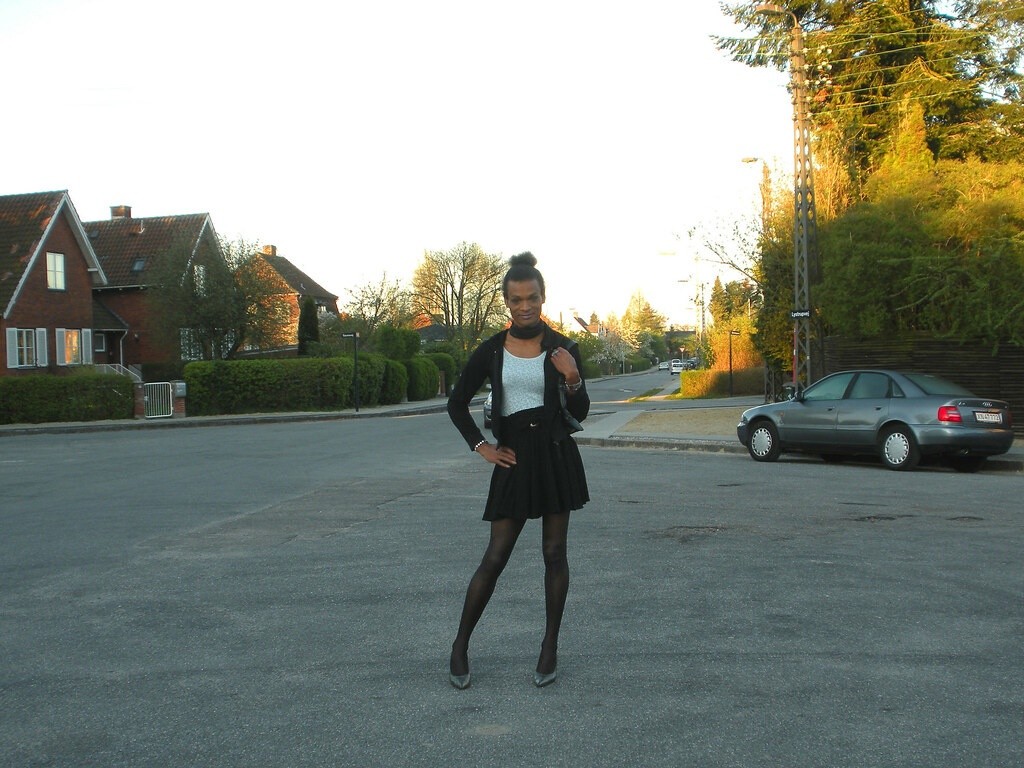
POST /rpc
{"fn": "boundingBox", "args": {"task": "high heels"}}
[534,646,557,686]
[449,647,470,688]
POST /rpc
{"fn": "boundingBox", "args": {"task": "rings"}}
[551,352,556,356]
[555,350,559,354]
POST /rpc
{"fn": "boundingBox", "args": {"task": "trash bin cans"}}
[781,382,795,401]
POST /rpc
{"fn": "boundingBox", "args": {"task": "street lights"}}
[755,5,816,385]
[729,330,741,397]
[677,279,706,369]
[342,332,359,412]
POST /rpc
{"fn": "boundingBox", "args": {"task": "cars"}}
[737,367,1015,469]
[659,361,669,371]
[484,384,494,430]
[671,356,701,375]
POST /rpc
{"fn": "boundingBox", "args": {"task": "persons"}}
[446,252,592,689]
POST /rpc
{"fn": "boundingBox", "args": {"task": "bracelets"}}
[474,440,486,451]
[565,377,582,392]
